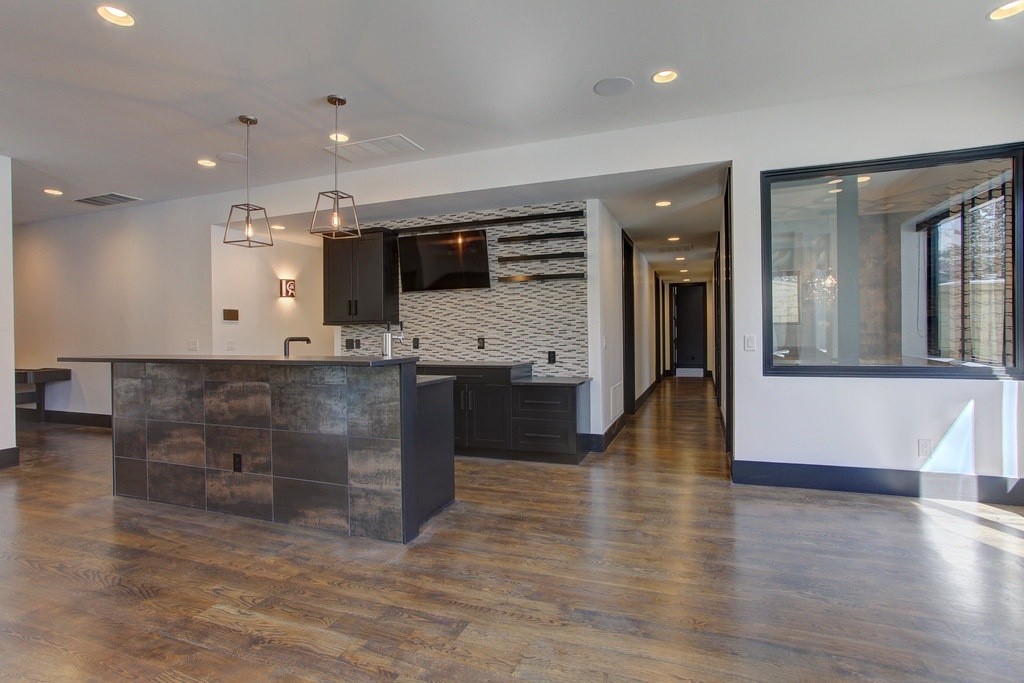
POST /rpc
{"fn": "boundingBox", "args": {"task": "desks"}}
[15,368,72,421]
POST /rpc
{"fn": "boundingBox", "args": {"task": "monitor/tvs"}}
[398,229,491,292]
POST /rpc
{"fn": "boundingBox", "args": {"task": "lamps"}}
[310,94,361,241]
[222,115,273,249]
[279,279,296,298]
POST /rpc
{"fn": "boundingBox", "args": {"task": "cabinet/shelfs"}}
[322,227,400,326]
[417,360,594,465]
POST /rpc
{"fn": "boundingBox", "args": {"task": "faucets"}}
[381,331,405,356]
[284,336,312,355]
